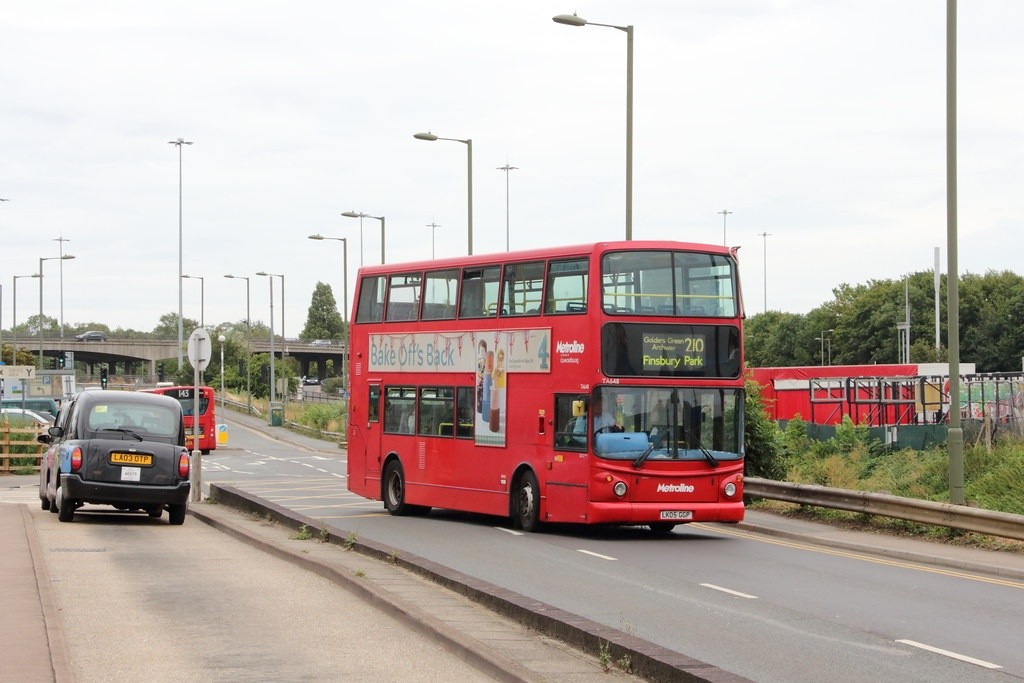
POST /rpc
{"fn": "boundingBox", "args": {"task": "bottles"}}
[538,409,545,434]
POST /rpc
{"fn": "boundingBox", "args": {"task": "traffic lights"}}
[49,357,57,370]
[59,350,66,367]
[156,364,163,381]
[100,367,108,387]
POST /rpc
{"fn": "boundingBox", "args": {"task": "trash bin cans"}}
[270,401,284,427]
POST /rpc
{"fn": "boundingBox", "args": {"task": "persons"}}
[374,392,392,420]
[408,401,428,434]
[572,395,624,447]
[442,406,472,437]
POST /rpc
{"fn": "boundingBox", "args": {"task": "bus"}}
[346,239,749,534]
[134,381,217,455]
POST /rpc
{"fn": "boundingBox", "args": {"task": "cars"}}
[311,340,332,348]
[49,390,191,526]
[75,331,107,343]
[303,377,319,385]
[0,399,60,440]
[36,400,73,512]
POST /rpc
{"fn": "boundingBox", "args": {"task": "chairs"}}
[376,302,705,321]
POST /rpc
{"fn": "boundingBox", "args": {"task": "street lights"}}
[413,131,476,256]
[341,211,386,305]
[551,13,634,308]
[224,274,253,417]
[181,273,205,329]
[168,135,194,377]
[255,272,286,401]
[218,336,226,417]
[39,254,75,371]
[495,163,521,253]
[53,237,70,340]
[814,337,830,366]
[424,221,441,258]
[308,235,349,399]
[820,329,835,366]
[11,272,45,366]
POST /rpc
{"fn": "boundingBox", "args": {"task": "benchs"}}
[399,404,413,433]
[430,406,448,435]
